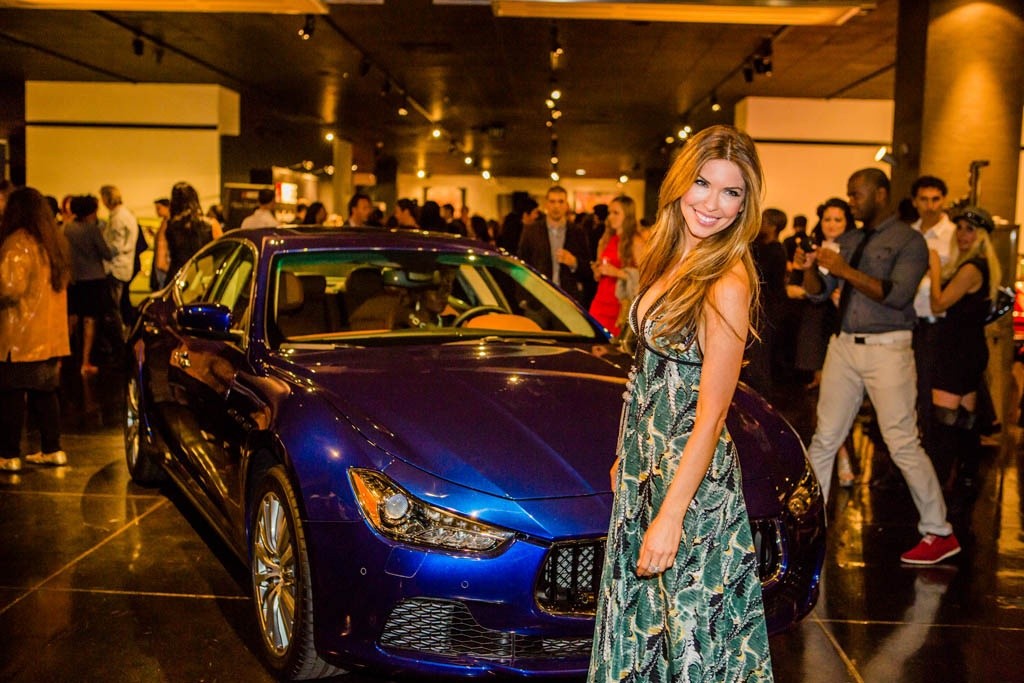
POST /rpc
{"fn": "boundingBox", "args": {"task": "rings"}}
[648,566,658,573]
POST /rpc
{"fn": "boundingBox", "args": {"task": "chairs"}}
[278,267,385,337]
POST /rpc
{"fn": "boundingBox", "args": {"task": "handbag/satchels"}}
[982,285,1017,330]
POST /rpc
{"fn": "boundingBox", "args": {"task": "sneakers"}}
[899,534,962,565]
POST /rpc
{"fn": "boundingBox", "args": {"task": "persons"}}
[0,167,1024,565]
[583,124,775,683]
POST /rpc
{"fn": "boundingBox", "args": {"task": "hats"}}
[817,198,854,228]
[950,206,994,234]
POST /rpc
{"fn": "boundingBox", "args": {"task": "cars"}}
[120,225,825,683]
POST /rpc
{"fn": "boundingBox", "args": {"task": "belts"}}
[852,338,867,344]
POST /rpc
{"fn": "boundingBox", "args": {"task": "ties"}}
[832,232,874,339]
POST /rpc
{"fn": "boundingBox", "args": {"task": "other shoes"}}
[0,456,23,474]
[23,450,68,467]
[79,364,99,377]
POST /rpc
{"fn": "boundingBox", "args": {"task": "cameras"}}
[800,238,815,253]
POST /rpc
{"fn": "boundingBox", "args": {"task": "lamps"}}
[743,39,773,83]
[876,147,898,167]
